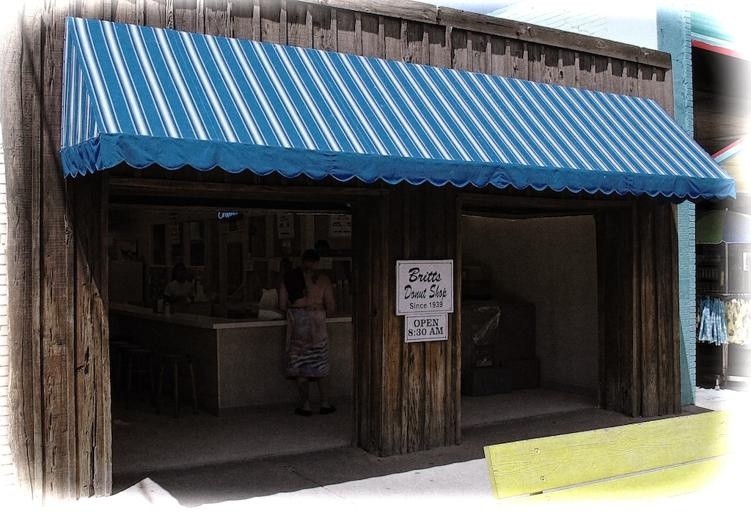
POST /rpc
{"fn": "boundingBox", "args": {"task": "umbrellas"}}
[694,206,751,368]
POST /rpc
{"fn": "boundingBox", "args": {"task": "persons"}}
[314,239,346,288]
[278,247,339,419]
[163,262,196,304]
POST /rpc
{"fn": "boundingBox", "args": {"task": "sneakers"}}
[320,403,338,414]
[294,404,313,416]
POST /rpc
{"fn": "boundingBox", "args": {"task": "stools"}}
[110,337,200,419]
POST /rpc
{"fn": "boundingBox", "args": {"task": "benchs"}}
[483,410,728,501]
